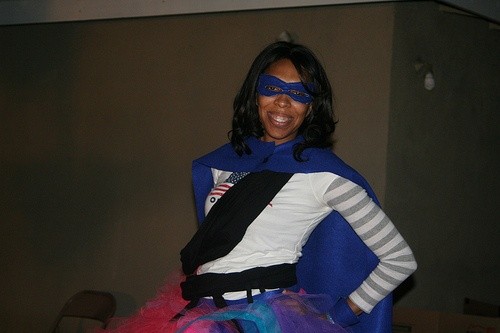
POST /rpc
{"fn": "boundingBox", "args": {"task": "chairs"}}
[49,289,117,333]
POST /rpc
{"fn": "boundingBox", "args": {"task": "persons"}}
[178,39,417,333]
